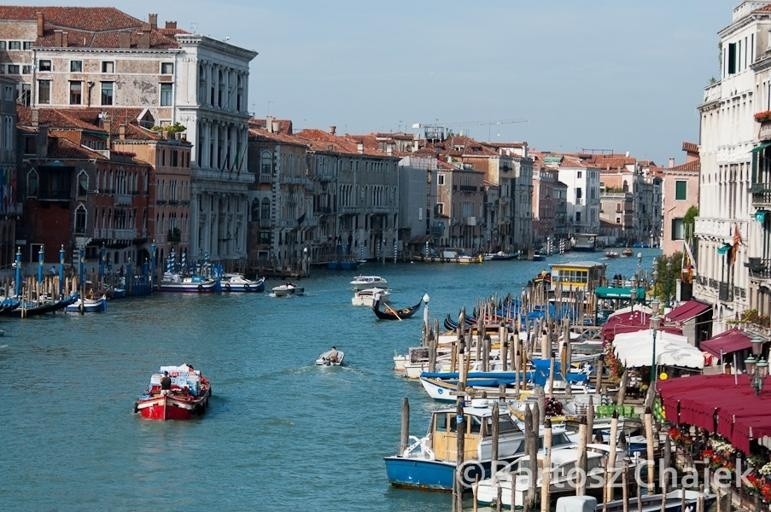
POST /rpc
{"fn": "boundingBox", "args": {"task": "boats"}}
[328,259,367,270]
[153,272,265,293]
[606,248,619,257]
[457,251,545,261]
[622,248,632,256]
[0,280,126,313]
[271,286,304,296]
[135,362,211,422]
[315,350,344,366]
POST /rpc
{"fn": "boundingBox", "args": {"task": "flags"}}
[680,223,744,284]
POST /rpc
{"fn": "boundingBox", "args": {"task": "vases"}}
[758,123,771,142]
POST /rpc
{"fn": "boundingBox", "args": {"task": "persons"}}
[327,346,339,363]
[160,370,171,398]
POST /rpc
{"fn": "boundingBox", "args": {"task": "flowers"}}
[754,111,771,123]
